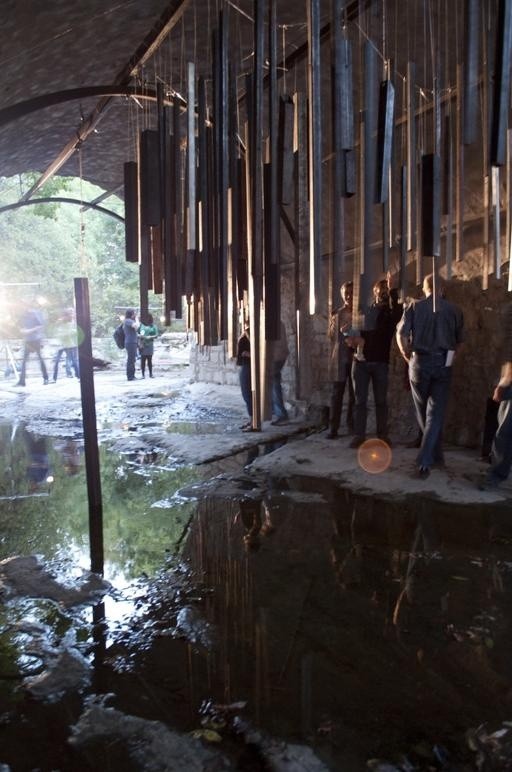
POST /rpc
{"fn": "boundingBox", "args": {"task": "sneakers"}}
[355,353,366,361]
[476,457,491,464]
[419,457,444,479]
[271,416,289,425]
[326,428,423,448]
[239,422,261,432]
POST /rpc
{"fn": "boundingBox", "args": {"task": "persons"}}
[13,303,50,387]
[395,271,465,479]
[462,293,512,489]
[136,311,157,379]
[325,270,405,451]
[122,307,143,380]
[237,308,289,433]
[49,307,81,385]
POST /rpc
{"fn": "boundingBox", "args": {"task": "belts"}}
[412,351,432,356]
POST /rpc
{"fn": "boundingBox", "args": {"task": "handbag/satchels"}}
[114,323,125,349]
